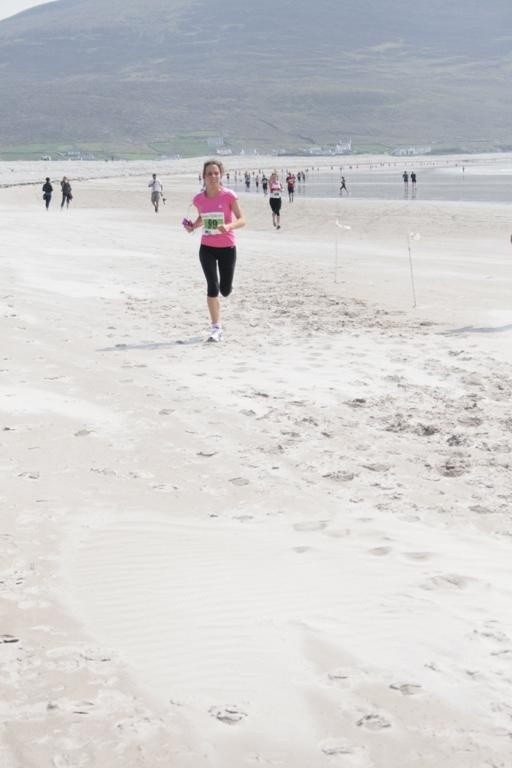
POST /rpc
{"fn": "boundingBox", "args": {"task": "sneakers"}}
[208,327,223,342]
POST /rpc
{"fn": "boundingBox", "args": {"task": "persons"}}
[184,160,247,342]
[42,175,54,210]
[59,174,72,210]
[148,172,164,213]
[197,166,417,232]
